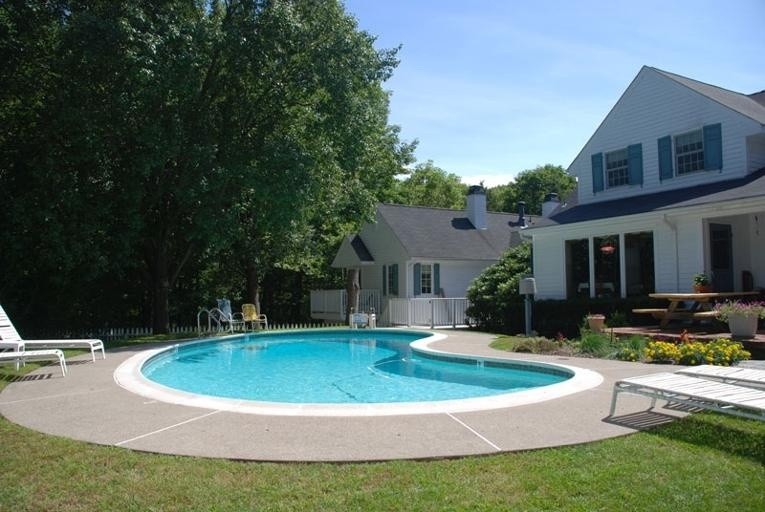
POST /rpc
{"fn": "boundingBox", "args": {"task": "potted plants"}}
[693,270,715,293]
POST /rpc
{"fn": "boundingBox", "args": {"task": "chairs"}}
[217,299,246,334]
[0,304,106,363]
[0,349,67,377]
[675,365,765,388]
[609,373,765,423]
[242,304,268,331]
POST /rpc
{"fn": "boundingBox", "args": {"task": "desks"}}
[648,291,760,327]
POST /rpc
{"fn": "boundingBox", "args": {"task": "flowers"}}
[713,299,765,323]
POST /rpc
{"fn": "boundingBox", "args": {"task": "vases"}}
[728,312,759,338]
[588,314,606,330]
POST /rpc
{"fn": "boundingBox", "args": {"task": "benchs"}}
[632,308,690,327]
[694,311,716,319]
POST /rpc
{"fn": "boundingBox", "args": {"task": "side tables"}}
[0,340,25,371]
